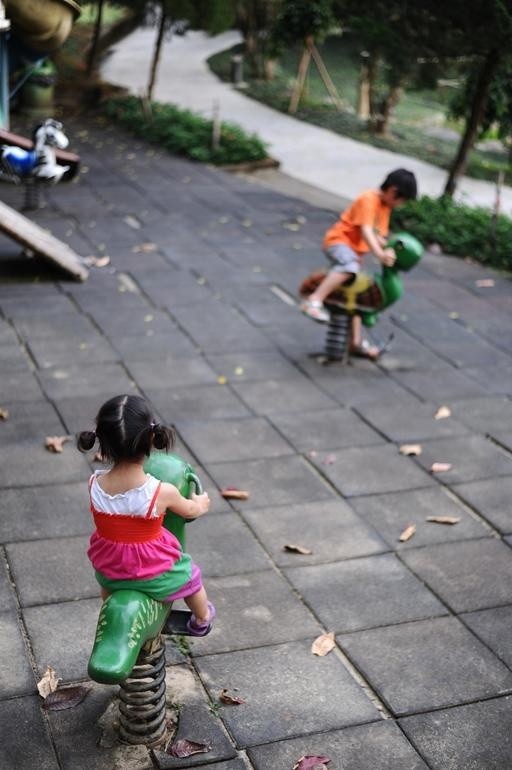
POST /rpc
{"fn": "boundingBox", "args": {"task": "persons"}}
[298,168,418,360]
[76,392,217,637]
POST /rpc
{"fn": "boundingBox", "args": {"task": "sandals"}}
[299,298,331,324]
[350,338,379,359]
[187,600,216,636]
